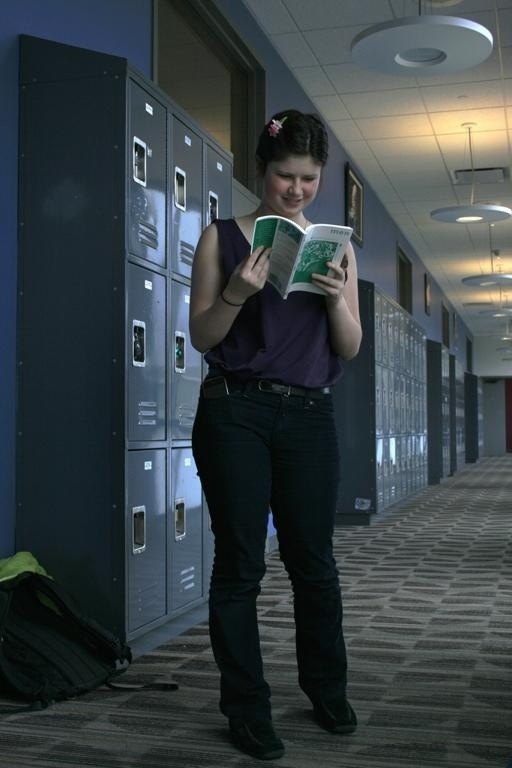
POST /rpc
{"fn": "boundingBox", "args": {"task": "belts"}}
[236,376,330,403]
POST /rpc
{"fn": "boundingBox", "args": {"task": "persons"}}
[189,110,361,761]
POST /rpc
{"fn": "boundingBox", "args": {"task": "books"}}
[249,216,352,299]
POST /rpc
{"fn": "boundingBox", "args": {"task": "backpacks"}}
[0,551,133,711]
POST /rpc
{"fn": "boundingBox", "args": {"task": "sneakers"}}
[313,701,358,734]
[229,720,285,761]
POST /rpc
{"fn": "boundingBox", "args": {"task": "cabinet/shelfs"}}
[12,30,237,645]
[331,279,485,518]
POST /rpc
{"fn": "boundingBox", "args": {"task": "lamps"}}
[428,120,511,321]
[349,1,497,80]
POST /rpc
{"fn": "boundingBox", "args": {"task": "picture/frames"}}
[343,160,364,249]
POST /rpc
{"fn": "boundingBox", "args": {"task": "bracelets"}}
[220,291,247,307]
